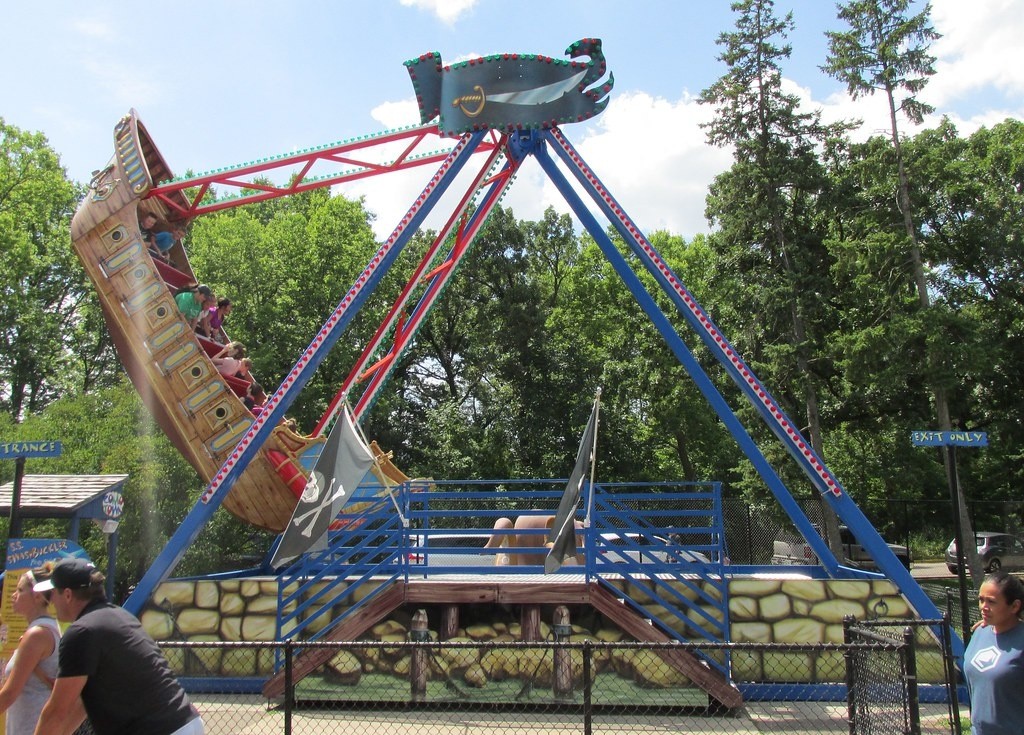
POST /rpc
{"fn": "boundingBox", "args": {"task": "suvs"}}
[769,525,912,573]
[592,532,710,564]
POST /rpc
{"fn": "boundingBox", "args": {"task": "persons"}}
[717,551,729,565]
[665,525,681,564]
[33,557,203,735]
[963,570,1024,735]
[195,297,231,339]
[211,341,246,377]
[239,382,264,411]
[151,225,187,261]
[252,394,273,415]
[197,293,215,343]
[139,212,158,247]
[234,358,252,380]
[0,561,62,735]
[173,282,211,332]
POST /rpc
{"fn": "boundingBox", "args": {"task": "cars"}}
[944,531,1024,574]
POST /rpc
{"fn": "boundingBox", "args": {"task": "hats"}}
[33,557,103,592]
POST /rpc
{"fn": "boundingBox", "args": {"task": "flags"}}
[544,398,601,574]
[270,404,375,570]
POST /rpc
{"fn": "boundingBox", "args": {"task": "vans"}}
[392,532,501,567]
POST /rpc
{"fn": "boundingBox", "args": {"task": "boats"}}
[71,107,434,535]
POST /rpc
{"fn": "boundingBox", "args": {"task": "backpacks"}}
[33,624,94,735]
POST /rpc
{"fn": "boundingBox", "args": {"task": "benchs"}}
[152,255,265,420]
